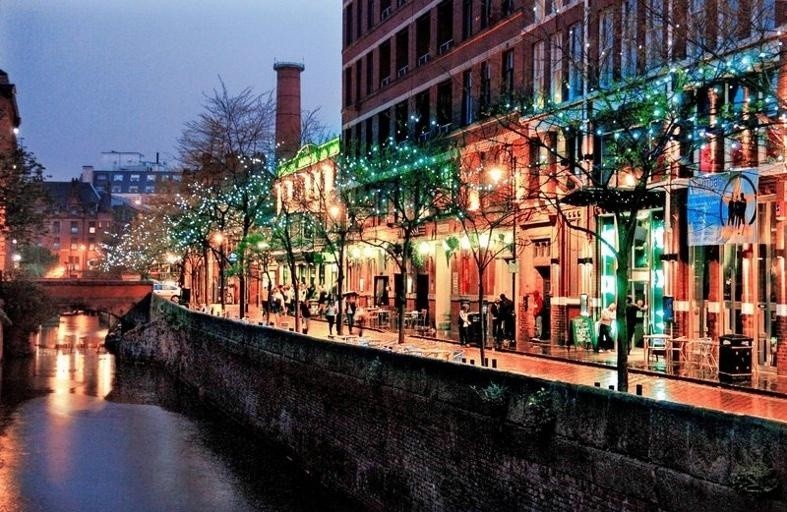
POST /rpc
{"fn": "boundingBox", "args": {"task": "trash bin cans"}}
[465,312,487,347]
[180,288,191,309]
[718,334,754,388]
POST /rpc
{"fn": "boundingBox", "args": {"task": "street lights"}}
[80,244,86,275]
[490,149,517,350]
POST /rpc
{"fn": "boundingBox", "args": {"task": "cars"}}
[153,283,182,304]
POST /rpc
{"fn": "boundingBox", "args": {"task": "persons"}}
[258,279,312,334]
[316,291,366,338]
[491,294,515,346]
[458,299,489,348]
[723,190,746,236]
[380,290,389,324]
[593,297,648,354]
[532,290,544,341]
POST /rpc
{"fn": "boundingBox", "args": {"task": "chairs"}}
[364,307,427,332]
[345,336,464,364]
[643,334,759,382]
